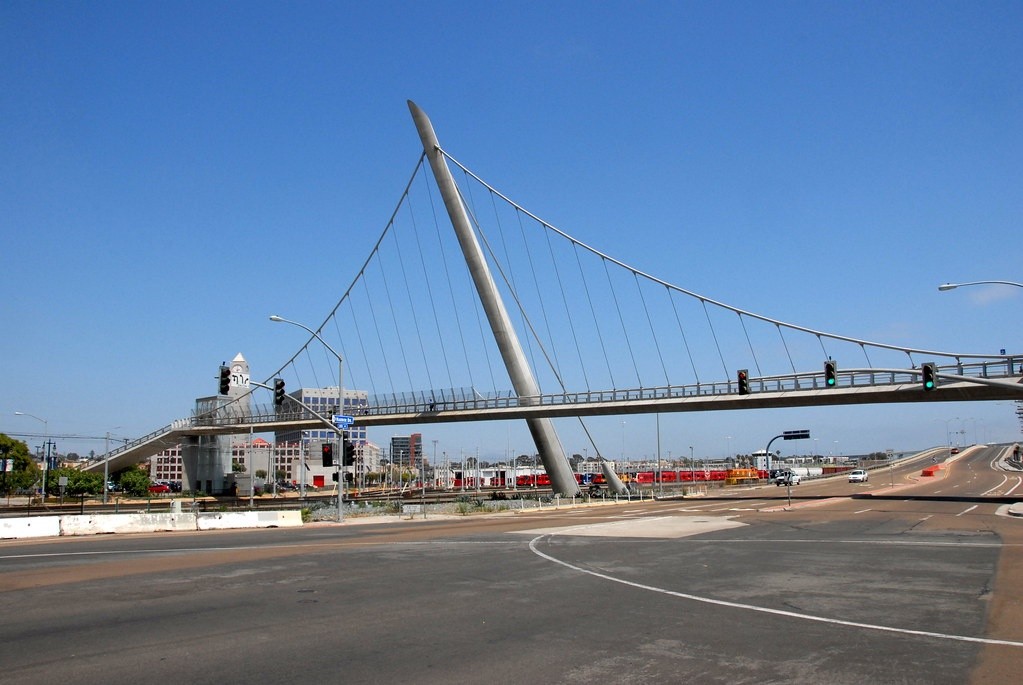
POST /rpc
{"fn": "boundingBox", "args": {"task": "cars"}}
[775,471,801,486]
[951,448,959,453]
[848,470,868,483]
[168,483,182,493]
[275,481,318,491]
[146,482,170,492]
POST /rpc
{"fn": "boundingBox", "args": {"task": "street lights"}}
[269,315,345,521]
[14,411,48,504]
[934,417,959,455]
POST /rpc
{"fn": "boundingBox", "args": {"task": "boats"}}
[737,369,750,394]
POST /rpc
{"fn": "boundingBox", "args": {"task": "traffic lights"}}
[274,380,286,405]
[922,362,937,392]
[218,366,231,397]
[824,360,838,388]
